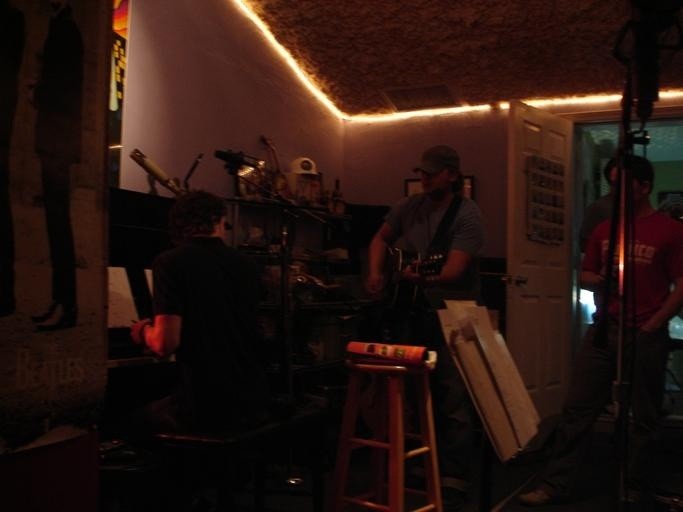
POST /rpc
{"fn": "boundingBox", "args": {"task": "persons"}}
[517,154,682,505]
[24,0,85,332]
[0,6,27,318]
[128,189,272,448]
[364,145,484,511]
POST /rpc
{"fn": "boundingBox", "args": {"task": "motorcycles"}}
[489,129,682,512]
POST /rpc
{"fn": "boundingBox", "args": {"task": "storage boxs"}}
[154,390,331,511]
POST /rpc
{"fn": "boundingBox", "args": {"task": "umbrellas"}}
[33,301,77,330]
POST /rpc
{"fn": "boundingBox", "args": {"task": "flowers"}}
[131,149,178,194]
[260,134,282,175]
[625,0,664,122]
[214,150,256,167]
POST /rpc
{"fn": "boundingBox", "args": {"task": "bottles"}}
[412,145,460,176]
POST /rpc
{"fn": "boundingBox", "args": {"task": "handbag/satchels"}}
[138,322,151,339]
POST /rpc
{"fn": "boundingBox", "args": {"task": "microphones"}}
[330,350,443,512]
